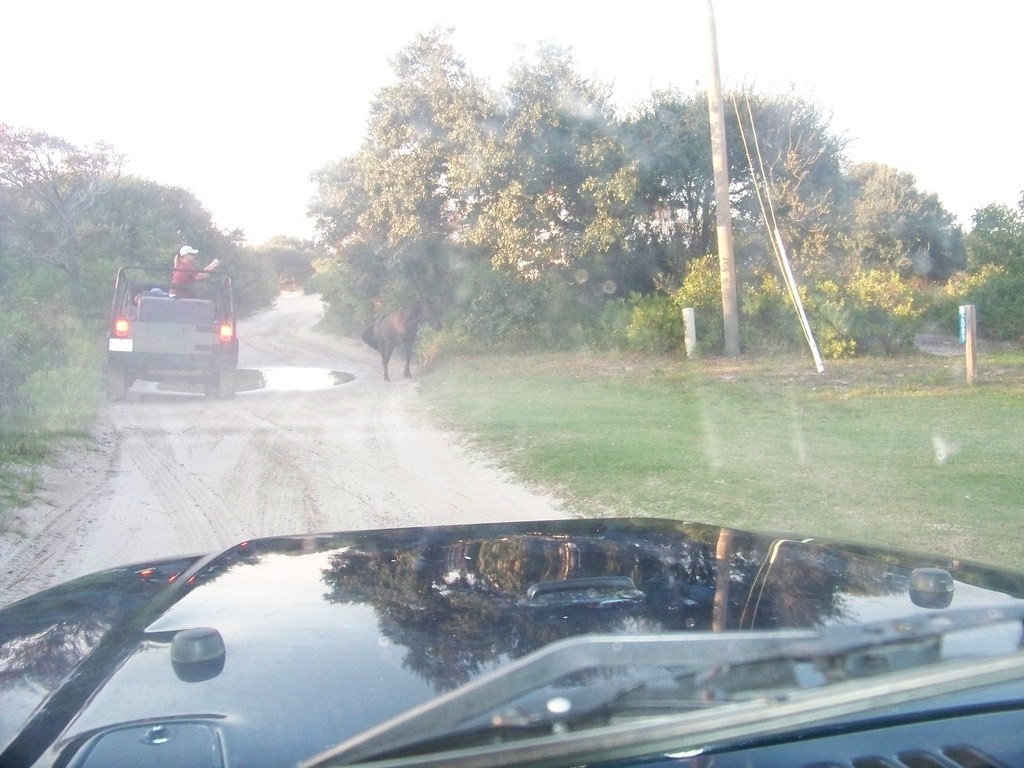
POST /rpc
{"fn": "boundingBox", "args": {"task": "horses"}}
[363,298,442,381]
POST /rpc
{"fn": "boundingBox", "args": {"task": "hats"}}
[151,288,169,297]
[180,246,199,256]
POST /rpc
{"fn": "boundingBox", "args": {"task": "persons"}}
[168,246,214,297]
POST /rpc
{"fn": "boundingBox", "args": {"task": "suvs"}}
[106,266,240,401]
